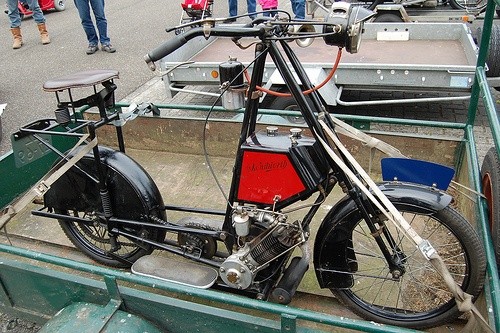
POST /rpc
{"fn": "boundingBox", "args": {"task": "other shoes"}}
[223,18,236,23]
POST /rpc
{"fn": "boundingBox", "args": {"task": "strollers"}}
[174,0,215,36]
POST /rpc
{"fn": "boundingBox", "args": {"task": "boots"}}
[37,21,51,45]
[10,26,24,49]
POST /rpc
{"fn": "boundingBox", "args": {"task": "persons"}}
[74,0,117,54]
[6,0,51,49]
[223,0,258,24]
[257,0,279,21]
[290,0,306,20]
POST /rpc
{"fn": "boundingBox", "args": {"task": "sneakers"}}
[86,44,99,54]
[101,42,116,53]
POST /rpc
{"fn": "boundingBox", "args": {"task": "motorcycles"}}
[10,0,488,331]
